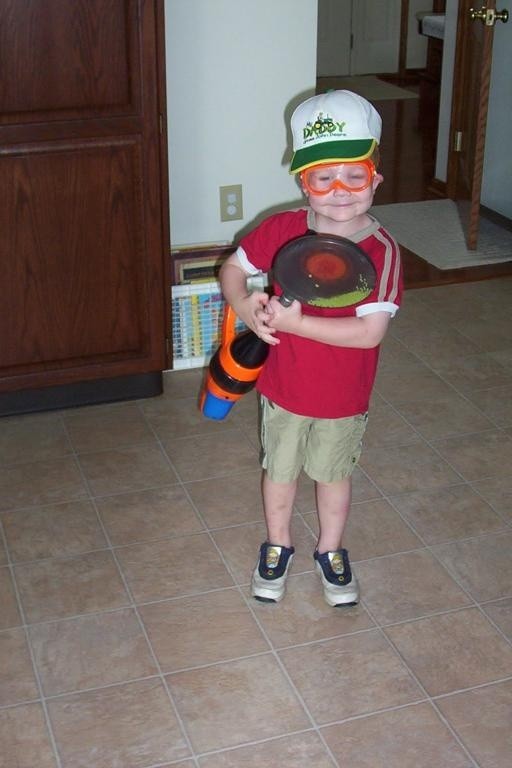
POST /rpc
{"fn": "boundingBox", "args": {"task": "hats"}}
[288,89,383,175]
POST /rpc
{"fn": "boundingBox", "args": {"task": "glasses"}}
[299,160,376,195]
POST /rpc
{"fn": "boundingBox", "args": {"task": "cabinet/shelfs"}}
[0,0,173,418]
[317,1,406,79]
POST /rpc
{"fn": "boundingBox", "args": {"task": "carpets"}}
[366,197,512,270]
[315,73,421,103]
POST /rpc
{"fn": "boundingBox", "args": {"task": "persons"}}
[215,89,403,610]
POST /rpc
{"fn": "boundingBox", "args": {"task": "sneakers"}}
[251,544,294,602]
[314,545,360,608]
